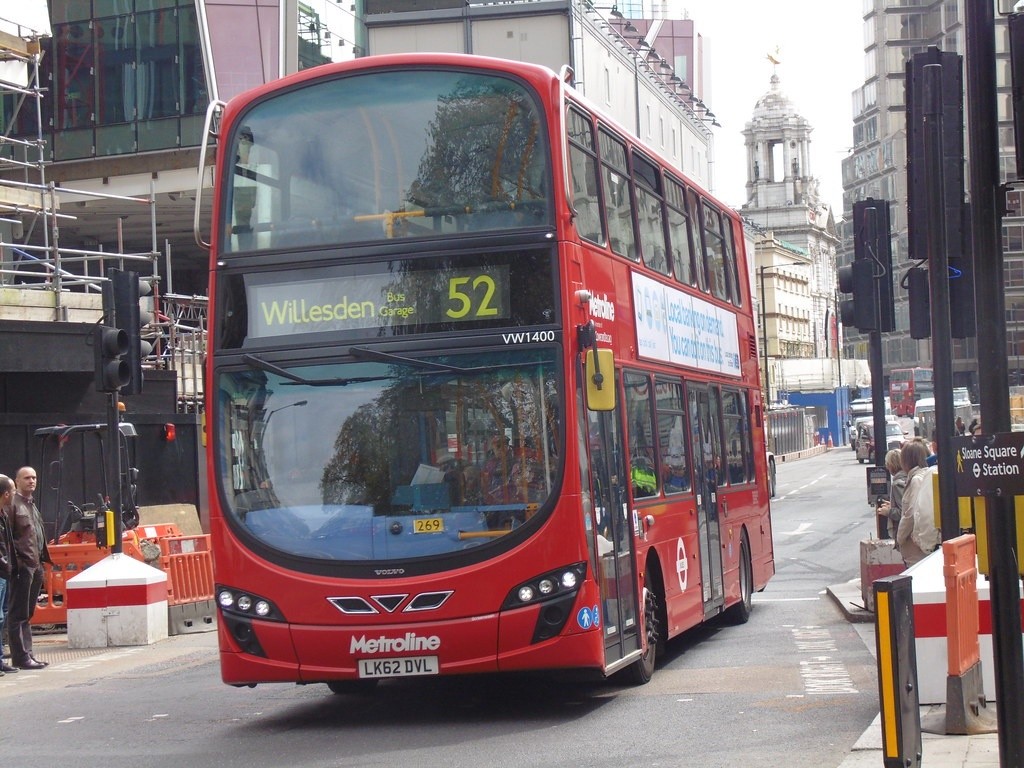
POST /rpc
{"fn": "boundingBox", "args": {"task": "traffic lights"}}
[94,324,132,391]
[837,260,859,329]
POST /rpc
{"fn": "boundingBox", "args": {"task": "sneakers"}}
[0,660,19,677]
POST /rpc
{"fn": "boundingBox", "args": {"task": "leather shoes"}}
[12,657,50,669]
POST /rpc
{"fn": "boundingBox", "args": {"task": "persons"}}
[876,416,982,565]
[0,466,51,678]
[593,443,745,557]
[457,436,557,530]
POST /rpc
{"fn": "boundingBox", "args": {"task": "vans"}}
[849,387,974,464]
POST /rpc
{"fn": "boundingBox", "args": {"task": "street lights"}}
[114,267,153,395]
[260,399,309,451]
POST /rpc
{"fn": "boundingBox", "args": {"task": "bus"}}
[889,367,934,419]
[192,52,776,696]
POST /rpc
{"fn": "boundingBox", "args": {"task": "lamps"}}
[623,36,649,47]
[594,6,623,17]
[701,118,721,127]
[685,99,707,108]
[657,72,682,81]
[694,109,715,117]
[647,58,673,70]
[636,48,659,59]
[610,21,638,31]
[676,93,698,102]
[666,82,691,91]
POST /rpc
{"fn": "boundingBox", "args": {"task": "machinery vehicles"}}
[15,401,219,637]
